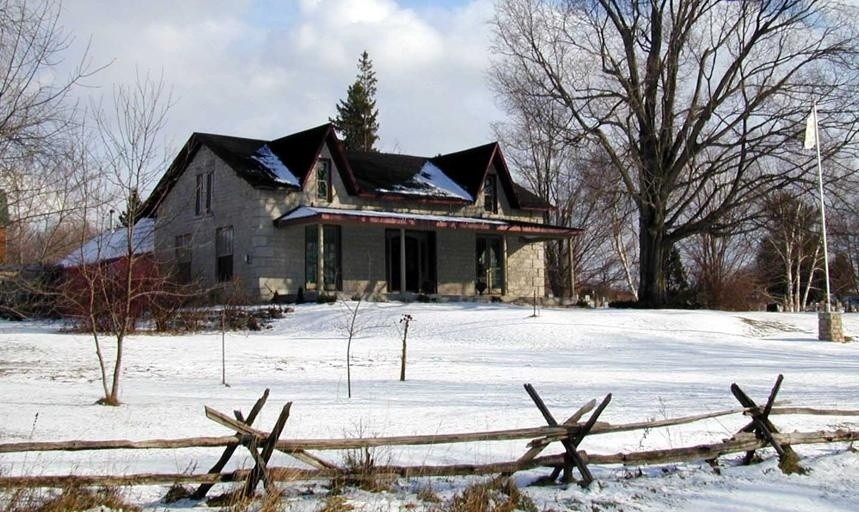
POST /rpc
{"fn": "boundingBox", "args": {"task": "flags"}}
[804,110,816,150]
